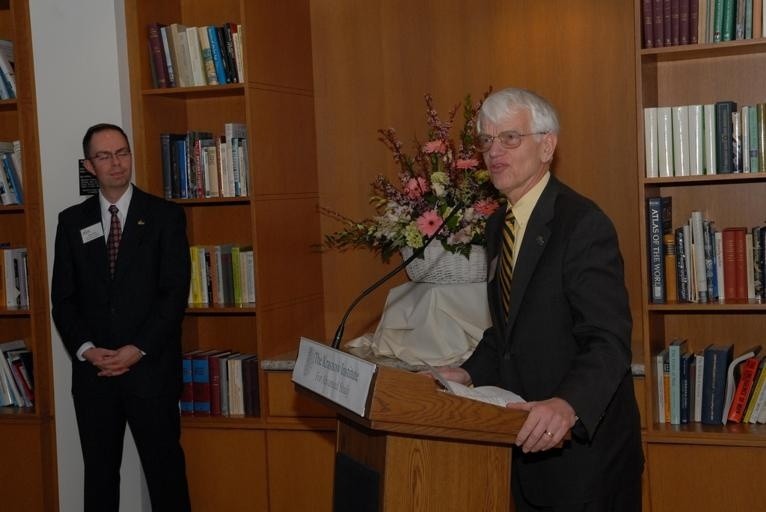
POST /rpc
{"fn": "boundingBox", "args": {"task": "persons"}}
[51,123,192,512]
[417,88,646,512]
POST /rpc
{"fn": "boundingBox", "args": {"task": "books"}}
[0,40,35,407]
[649,195,766,303]
[657,339,766,427]
[644,101,766,178]
[643,0,766,49]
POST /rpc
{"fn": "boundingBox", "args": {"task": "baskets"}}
[400,243,490,283]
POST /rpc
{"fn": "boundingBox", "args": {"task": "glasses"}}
[471,129,547,152]
[88,149,131,163]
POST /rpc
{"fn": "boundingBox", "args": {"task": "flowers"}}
[305,88,500,262]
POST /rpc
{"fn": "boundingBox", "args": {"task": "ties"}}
[106,205,122,279]
[499,207,516,322]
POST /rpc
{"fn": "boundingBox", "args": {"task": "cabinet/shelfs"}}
[630,3,766,437]
[0,1,59,509]
[114,2,317,422]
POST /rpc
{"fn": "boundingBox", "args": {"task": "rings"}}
[544,430,554,438]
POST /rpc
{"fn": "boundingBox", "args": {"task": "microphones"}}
[331,200,464,349]
